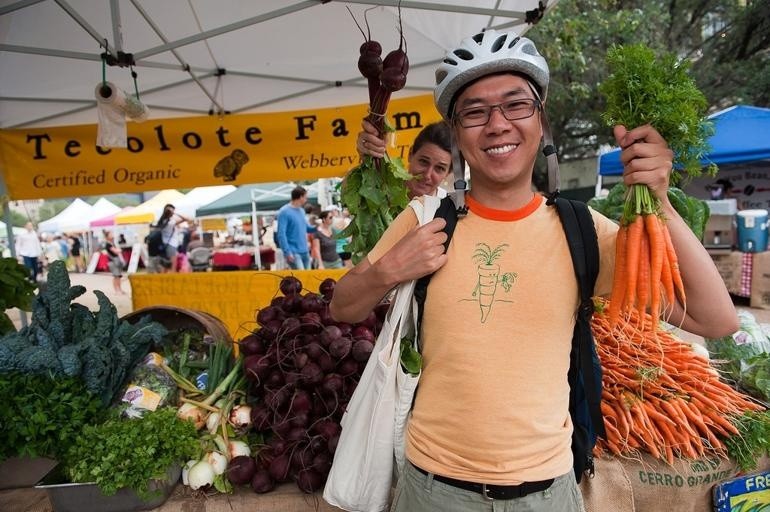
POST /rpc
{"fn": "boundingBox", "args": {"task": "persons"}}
[355,119,455,201]
[705,178,743,212]
[327,26,746,511]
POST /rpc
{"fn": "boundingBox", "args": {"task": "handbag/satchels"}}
[318,284,428,511]
[113,258,127,267]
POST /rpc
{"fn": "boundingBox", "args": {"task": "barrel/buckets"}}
[737,208,768,252]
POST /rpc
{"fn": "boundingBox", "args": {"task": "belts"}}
[404,455,556,498]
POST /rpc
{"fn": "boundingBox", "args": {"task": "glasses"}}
[454,97,534,128]
[327,215,333,220]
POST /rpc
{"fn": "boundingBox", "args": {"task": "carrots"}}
[476,263,499,322]
[610,218,686,342]
[224,276,376,496]
[592,297,767,464]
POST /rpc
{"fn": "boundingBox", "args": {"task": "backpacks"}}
[145,231,166,257]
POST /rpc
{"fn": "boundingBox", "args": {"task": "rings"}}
[362,139,366,147]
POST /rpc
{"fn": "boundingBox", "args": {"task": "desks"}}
[210,243,276,272]
[705,248,769,310]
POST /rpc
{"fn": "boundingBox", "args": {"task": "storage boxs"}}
[701,214,737,247]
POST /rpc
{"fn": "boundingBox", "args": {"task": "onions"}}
[176,403,253,492]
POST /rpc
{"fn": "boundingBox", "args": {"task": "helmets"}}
[432,28,551,116]
